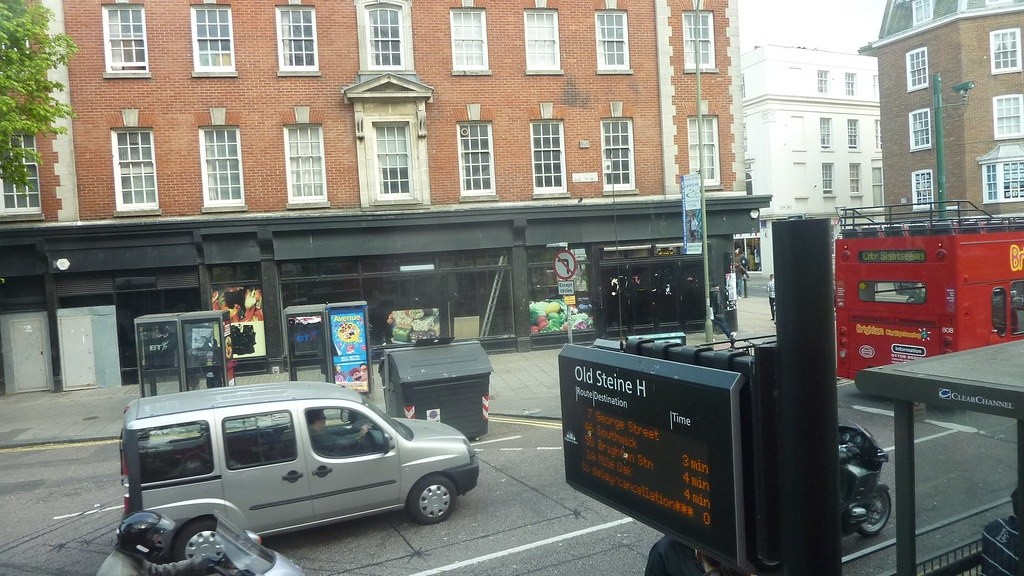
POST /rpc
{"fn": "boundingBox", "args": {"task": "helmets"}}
[116,511,176,557]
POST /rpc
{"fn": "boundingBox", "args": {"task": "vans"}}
[118,379,480,539]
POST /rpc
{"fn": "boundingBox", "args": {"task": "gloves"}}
[191,552,220,572]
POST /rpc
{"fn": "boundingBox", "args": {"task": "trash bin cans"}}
[378,338,492,440]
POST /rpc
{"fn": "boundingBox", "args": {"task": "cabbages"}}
[548,312,559,321]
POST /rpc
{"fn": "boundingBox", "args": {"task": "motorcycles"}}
[837,414,892,540]
[206,509,308,576]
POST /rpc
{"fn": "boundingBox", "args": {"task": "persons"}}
[709,283,737,341]
[198,333,223,387]
[767,274,777,321]
[645,532,744,576]
[735,262,750,299]
[310,410,369,456]
[96,510,225,576]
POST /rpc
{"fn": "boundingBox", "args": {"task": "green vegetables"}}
[530,298,590,334]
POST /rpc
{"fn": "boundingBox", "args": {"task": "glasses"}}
[315,416,327,420]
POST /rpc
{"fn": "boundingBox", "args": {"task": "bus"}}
[834,199,1024,380]
